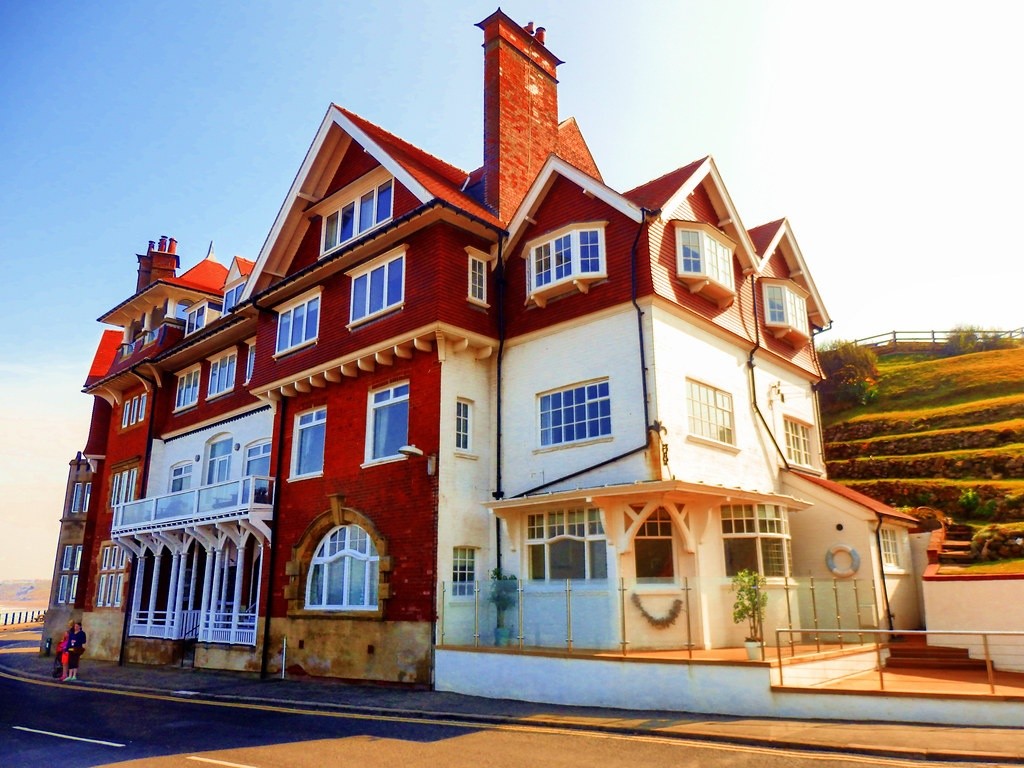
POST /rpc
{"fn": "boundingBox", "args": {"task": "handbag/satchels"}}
[73,646,85,655]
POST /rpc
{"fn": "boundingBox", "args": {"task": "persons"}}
[62,622,86,682]
[56,619,75,680]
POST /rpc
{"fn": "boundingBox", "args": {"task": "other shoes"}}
[72,676,77,680]
[63,677,72,681]
[57,675,66,680]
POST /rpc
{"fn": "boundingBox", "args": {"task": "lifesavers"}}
[825,543,861,578]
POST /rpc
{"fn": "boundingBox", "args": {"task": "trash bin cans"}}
[44,638,52,656]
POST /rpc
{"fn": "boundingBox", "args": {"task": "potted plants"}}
[491,568,519,647]
[731,570,768,659]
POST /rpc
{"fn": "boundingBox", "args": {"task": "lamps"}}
[398,446,436,476]
